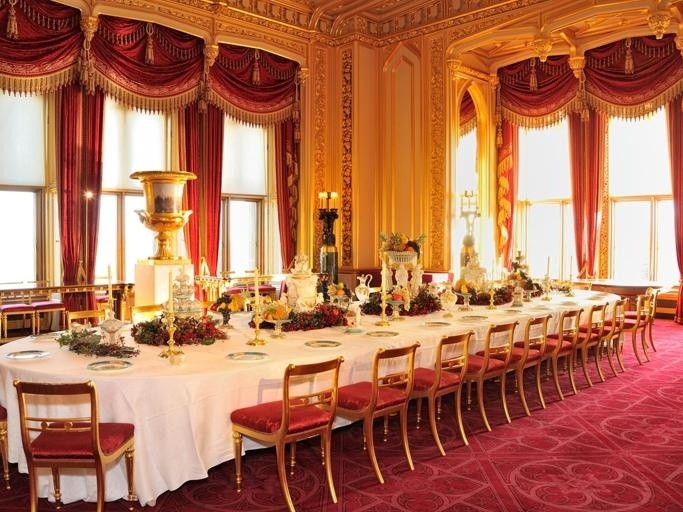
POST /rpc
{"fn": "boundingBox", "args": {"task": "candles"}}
[459,195,478,213]
[318,191,340,210]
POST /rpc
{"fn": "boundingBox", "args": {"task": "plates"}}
[86,360,133,374]
[5,350,50,360]
[366,331,400,337]
[459,316,488,322]
[423,322,452,329]
[226,352,272,363]
[305,340,343,350]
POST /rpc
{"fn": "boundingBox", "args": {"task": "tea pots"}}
[354,274,373,303]
